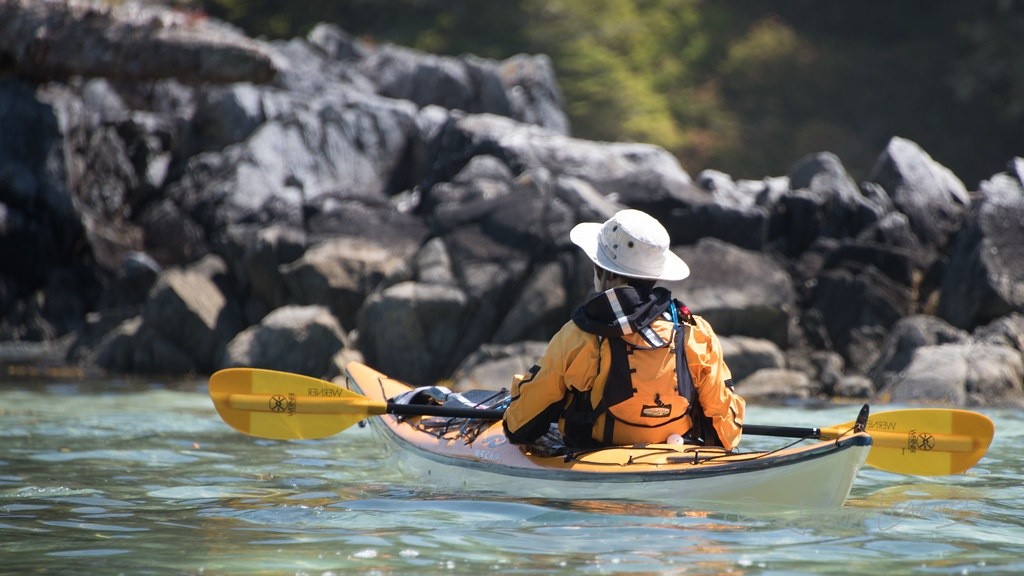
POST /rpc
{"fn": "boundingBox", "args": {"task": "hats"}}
[569,209,690,281]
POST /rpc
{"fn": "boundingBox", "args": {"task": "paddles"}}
[206,365,996,479]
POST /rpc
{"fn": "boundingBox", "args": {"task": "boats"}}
[341,361,870,512]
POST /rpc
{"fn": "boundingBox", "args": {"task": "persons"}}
[501,209,746,452]
